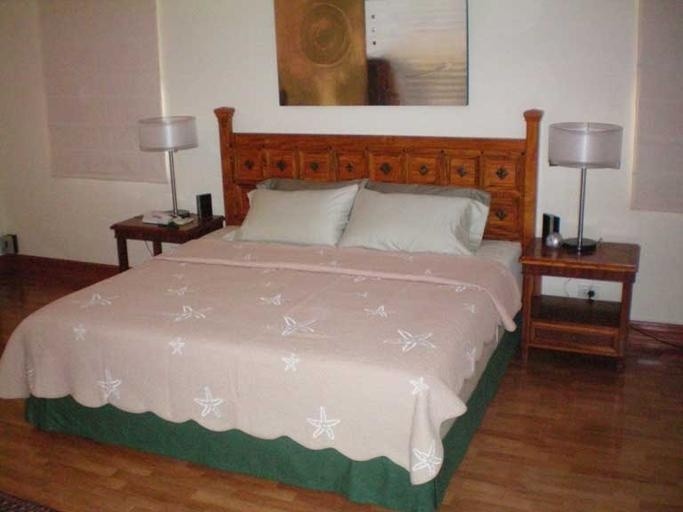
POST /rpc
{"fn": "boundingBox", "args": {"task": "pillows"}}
[339,181,492,256]
[221,176,368,246]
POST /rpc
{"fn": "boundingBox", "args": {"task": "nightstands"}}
[519,237,638,371]
[109,208,225,279]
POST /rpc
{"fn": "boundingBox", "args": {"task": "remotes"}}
[175,217,194,225]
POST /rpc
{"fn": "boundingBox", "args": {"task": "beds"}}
[0,107,543,512]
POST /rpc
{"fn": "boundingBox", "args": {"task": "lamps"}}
[546,123,622,254]
[135,116,199,215]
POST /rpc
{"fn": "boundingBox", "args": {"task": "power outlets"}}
[576,282,599,300]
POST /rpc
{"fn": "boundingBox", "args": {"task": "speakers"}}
[541,213,559,246]
[197,193,212,219]
